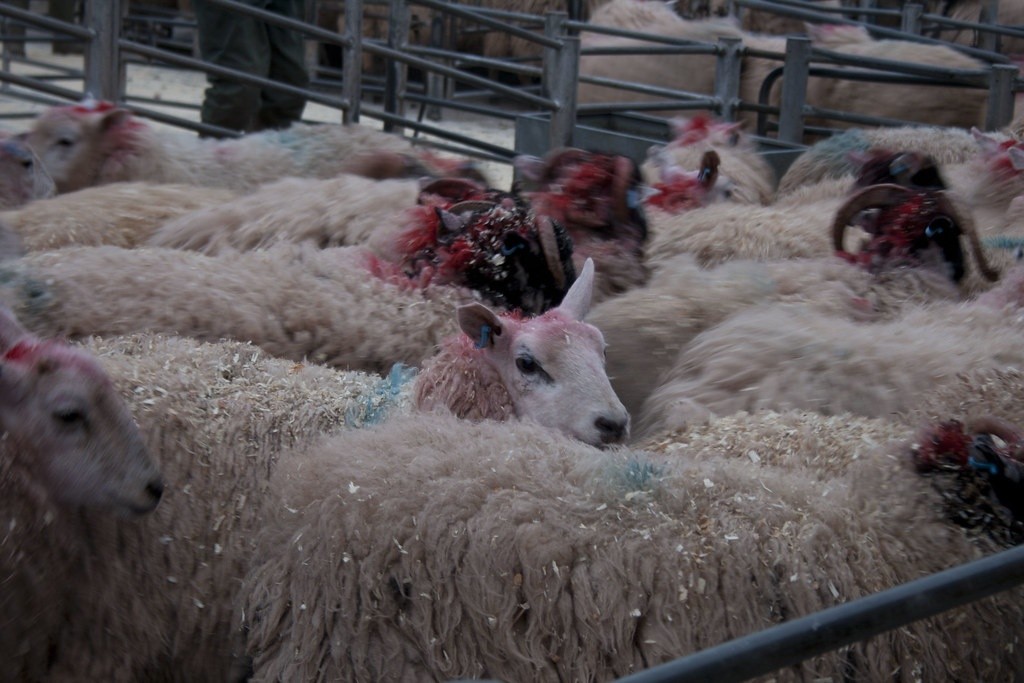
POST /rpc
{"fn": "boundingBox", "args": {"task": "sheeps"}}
[0,0,1024,683]
